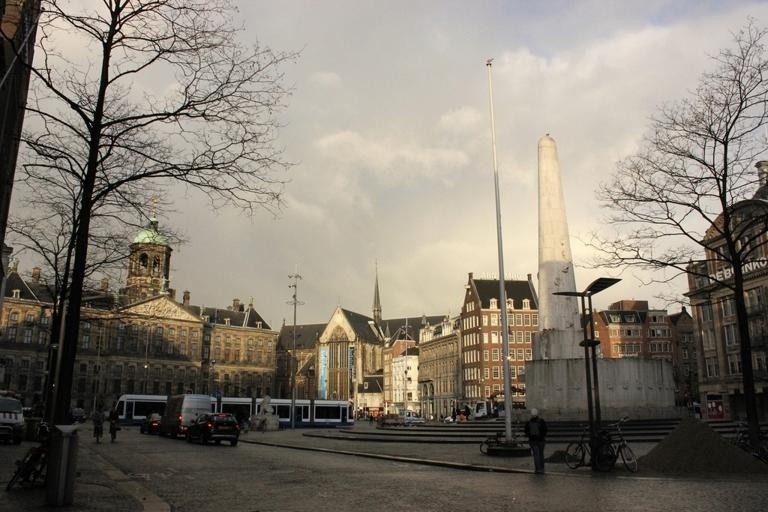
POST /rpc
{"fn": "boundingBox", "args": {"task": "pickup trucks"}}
[383,411,425,425]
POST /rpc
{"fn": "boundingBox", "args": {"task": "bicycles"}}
[564,424,615,469]
[591,417,638,473]
[479,432,523,455]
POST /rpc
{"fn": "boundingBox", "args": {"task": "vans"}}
[0,396,26,445]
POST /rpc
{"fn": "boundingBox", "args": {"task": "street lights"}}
[286,271,305,396]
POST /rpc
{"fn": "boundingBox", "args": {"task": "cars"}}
[140,410,163,435]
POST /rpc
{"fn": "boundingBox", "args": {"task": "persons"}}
[524,407,547,474]
[369,413,373,424]
[451,407,457,420]
[91,406,104,444]
[456,411,465,422]
[464,404,471,420]
[107,406,121,443]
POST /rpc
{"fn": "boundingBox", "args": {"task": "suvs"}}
[185,413,241,446]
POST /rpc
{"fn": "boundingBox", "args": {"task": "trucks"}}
[159,394,212,438]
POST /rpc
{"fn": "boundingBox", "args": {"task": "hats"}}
[531,408,538,415]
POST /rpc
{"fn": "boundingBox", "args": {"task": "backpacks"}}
[529,422,540,437]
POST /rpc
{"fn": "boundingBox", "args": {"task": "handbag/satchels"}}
[114,423,121,431]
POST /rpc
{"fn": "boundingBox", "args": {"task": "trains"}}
[114,393,217,425]
[220,396,354,425]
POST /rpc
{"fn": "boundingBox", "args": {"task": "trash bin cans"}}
[45,425,81,507]
